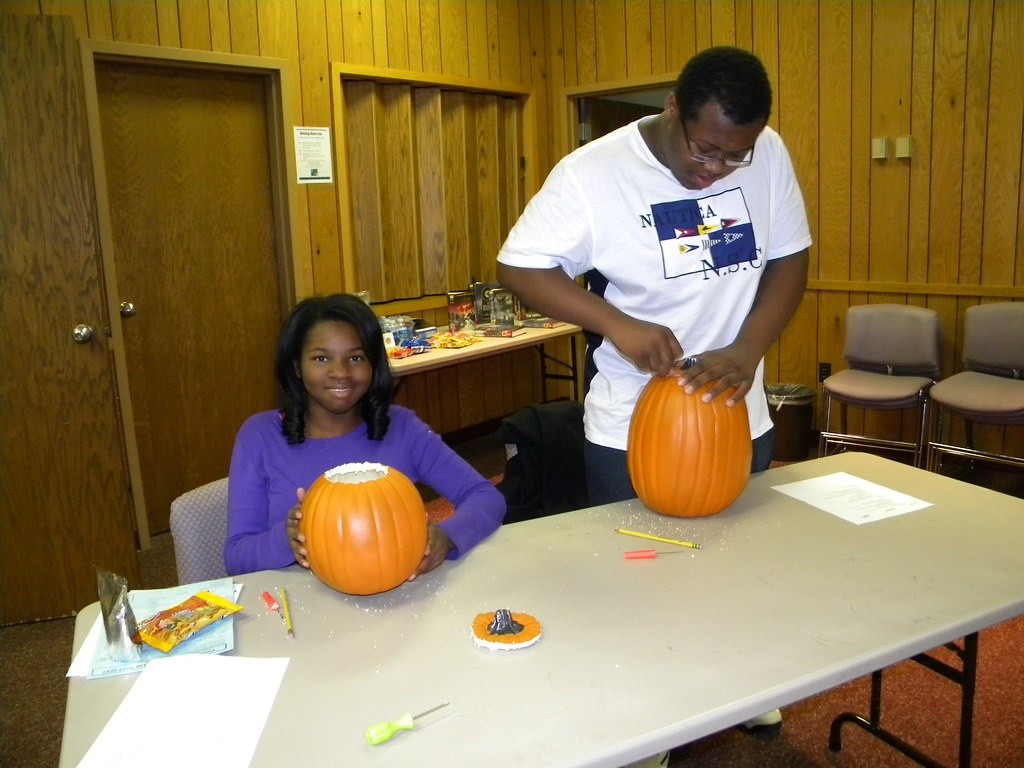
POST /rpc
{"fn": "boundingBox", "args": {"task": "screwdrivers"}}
[364,702,451,745]
[624,549,686,559]
[263,591,285,622]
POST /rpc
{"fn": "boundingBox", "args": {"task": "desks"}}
[61,451,1024,768]
[376,313,583,411]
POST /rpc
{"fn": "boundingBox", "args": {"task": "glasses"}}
[674,93,754,168]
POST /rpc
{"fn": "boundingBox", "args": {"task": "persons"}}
[223,292,507,582]
[495,45,813,768]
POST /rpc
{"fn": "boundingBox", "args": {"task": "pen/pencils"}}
[279,588,294,639]
[614,528,703,550]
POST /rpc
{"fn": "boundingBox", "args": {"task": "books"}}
[447,282,566,338]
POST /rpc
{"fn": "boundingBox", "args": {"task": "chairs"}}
[171,475,227,584]
[824,302,954,469]
[928,300,1024,492]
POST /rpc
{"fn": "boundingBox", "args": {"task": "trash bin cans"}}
[766,382,818,461]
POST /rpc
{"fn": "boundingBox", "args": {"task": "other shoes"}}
[632,749,670,768]
[741,707,782,734]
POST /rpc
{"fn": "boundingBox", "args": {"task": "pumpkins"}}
[627,357,753,517]
